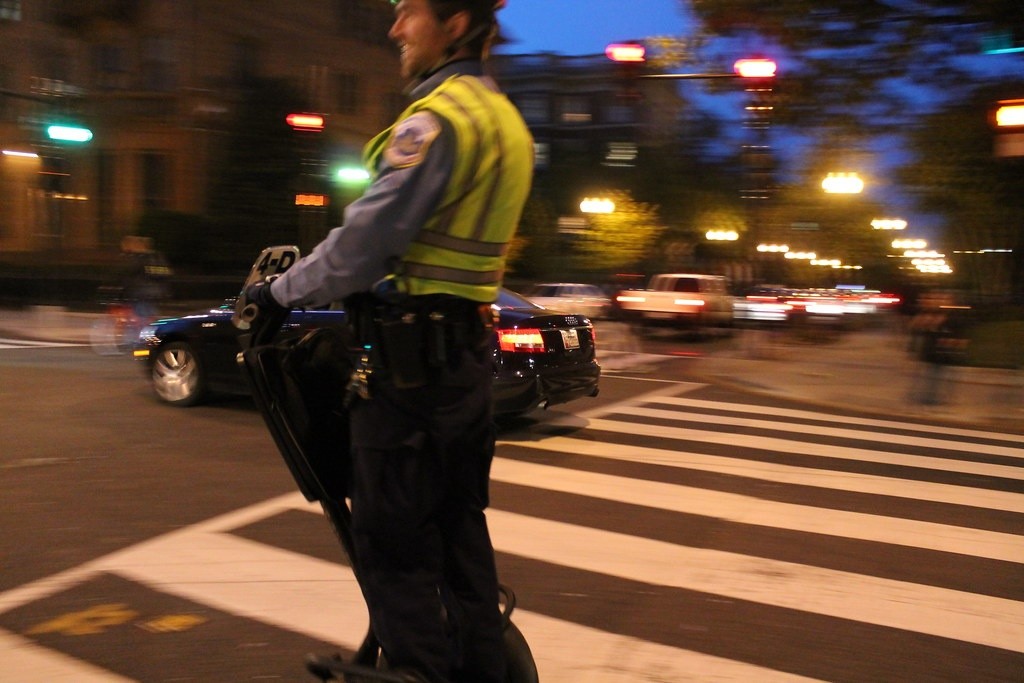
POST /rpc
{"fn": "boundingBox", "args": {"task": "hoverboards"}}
[218,242,539,683]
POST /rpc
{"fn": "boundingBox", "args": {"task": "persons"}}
[247,0,537,683]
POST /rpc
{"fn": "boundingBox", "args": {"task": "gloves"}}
[246,283,289,346]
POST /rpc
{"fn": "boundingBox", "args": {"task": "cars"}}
[519,280,614,325]
[133,270,601,427]
[618,273,734,328]
